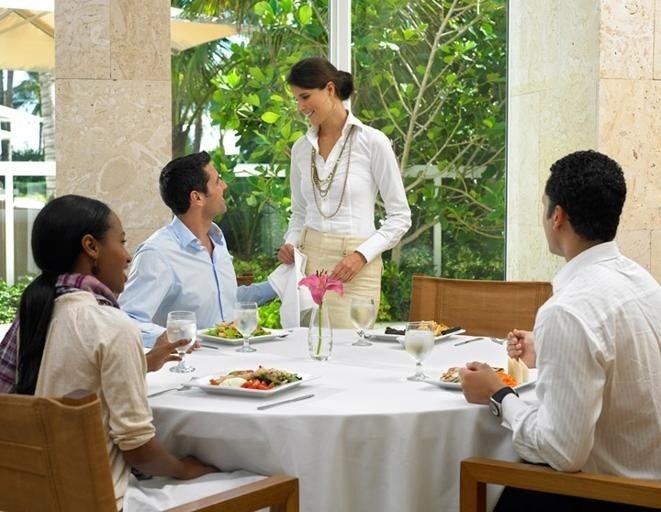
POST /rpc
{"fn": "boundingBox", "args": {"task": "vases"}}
[308,301,332,361]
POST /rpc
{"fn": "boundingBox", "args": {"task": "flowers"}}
[297,267,343,355]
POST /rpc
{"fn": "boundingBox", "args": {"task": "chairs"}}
[459,456,661,512]
[408,275,553,344]
[0,388,299,512]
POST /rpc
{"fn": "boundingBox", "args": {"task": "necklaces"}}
[309,125,355,220]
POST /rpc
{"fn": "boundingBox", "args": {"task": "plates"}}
[192,367,320,398]
[426,372,536,390]
[365,326,466,343]
[197,328,288,345]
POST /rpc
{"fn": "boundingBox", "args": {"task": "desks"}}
[149,325,538,512]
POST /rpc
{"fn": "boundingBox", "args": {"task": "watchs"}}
[488,386,520,418]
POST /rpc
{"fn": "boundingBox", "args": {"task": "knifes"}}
[256,392,316,411]
[454,337,484,347]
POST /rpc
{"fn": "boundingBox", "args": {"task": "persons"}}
[458,146,660,512]
[116,149,279,354]
[277,56,413,328]
[0,194,220,511]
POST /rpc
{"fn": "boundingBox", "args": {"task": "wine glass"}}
[166,310,198,375]
[234,302,258,354]
[404,322,434,383]
[351,298,376,346]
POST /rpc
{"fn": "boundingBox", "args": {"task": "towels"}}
[267,247,319,329]
